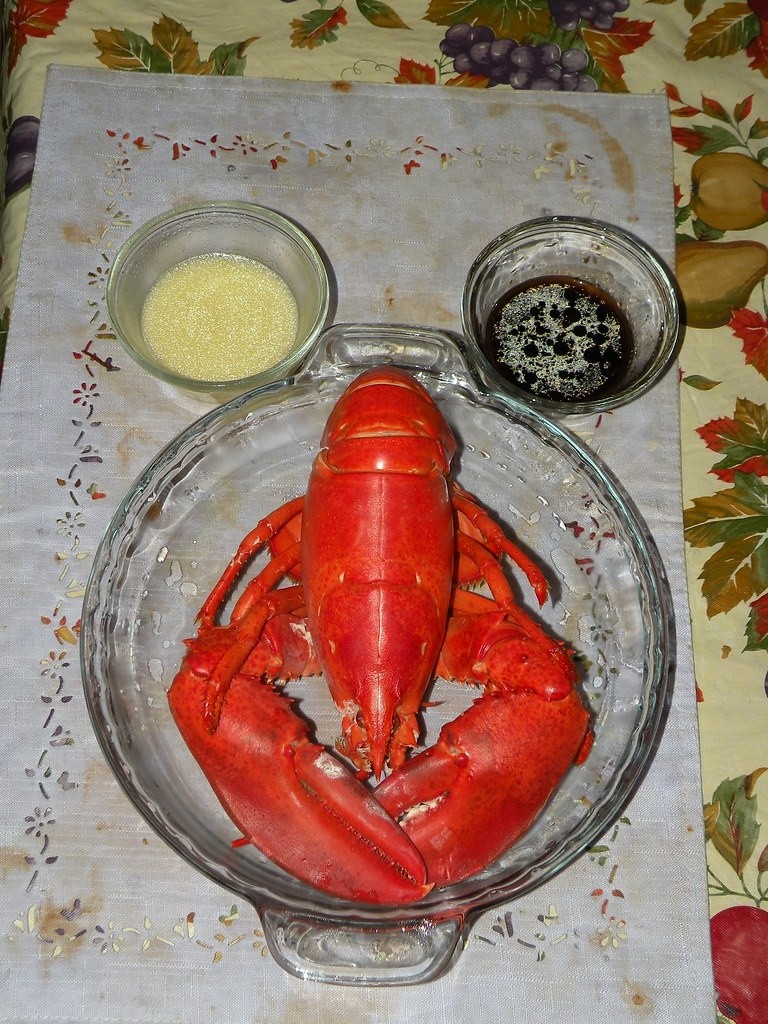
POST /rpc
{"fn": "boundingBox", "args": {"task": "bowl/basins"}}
[462,216,686,416]
[107,199,332,399]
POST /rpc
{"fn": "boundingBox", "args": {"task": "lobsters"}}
[166,364,595,905]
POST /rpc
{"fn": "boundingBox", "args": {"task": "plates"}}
[80,322,677,988]
